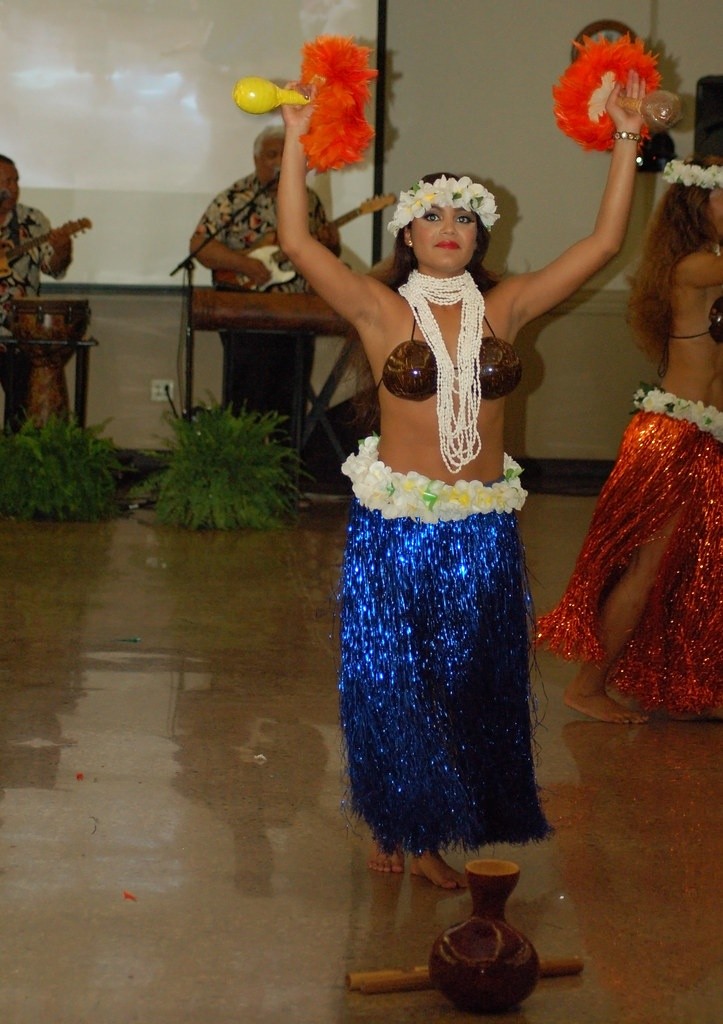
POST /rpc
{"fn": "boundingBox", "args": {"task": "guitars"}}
[211,192,398,293]
[0,216,94,278]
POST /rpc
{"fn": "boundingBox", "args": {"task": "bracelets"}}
[612,131,639,141]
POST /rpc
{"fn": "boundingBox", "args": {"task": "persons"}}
[0,156,73,436]
[277,68,649,890]
[190,126,341,448]
[537,156,723,725]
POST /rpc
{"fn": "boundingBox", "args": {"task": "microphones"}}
[273,165,281,181]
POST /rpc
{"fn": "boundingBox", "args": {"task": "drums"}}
[6,296,92,435]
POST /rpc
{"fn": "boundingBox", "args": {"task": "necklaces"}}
[398,268,486,473]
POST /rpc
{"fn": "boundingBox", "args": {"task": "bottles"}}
[428,861,538,1012]
